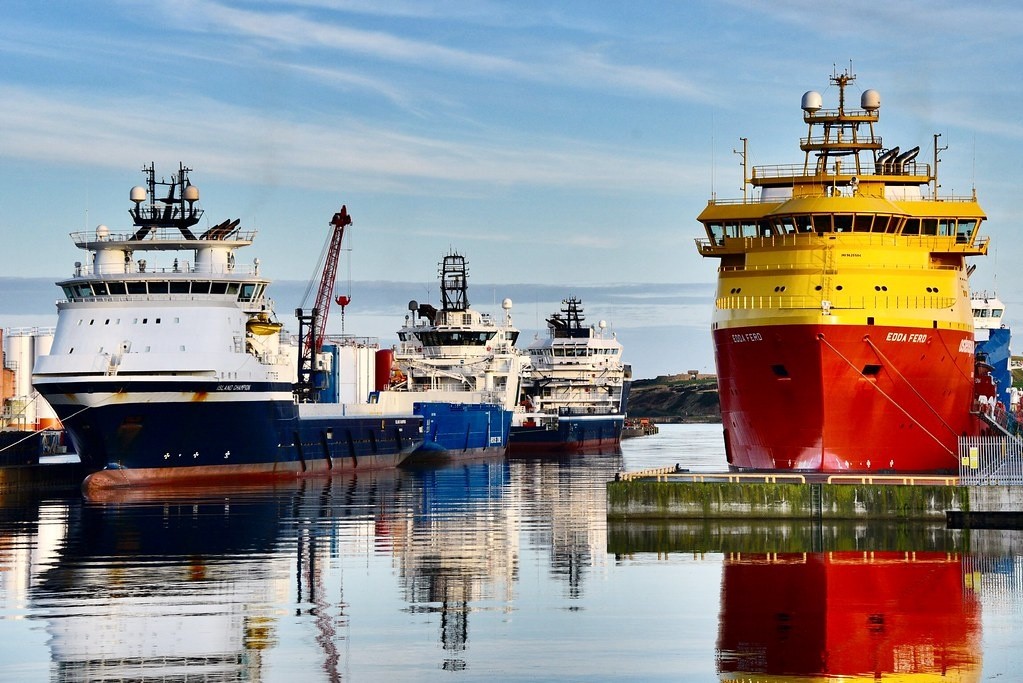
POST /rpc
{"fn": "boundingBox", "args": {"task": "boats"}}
[245,313,282,336]
[31,162,633,491]
[692,56,1023,475]
